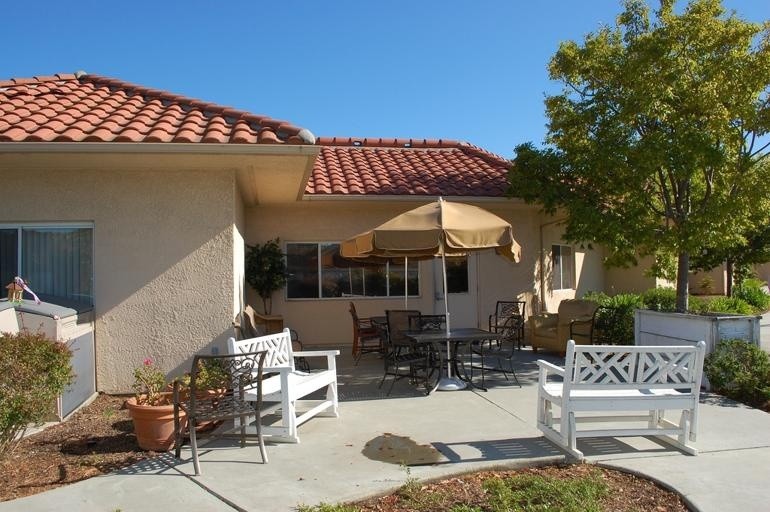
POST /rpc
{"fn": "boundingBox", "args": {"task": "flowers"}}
[130,356,164,404]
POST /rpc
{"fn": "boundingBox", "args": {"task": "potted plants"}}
[170,359,227,430]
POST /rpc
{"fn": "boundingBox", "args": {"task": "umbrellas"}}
[339,194,523,378]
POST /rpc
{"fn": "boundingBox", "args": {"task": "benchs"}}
[536,340,705,459]
[243,305,310,374]
[226,327,341,443]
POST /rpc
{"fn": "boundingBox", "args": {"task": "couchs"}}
[529,300,598,353]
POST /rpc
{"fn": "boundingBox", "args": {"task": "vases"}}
[125,395,189,449]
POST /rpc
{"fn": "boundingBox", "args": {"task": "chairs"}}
[349,299,525,395]
[568,306,618,345]
[173,350,267,475]
